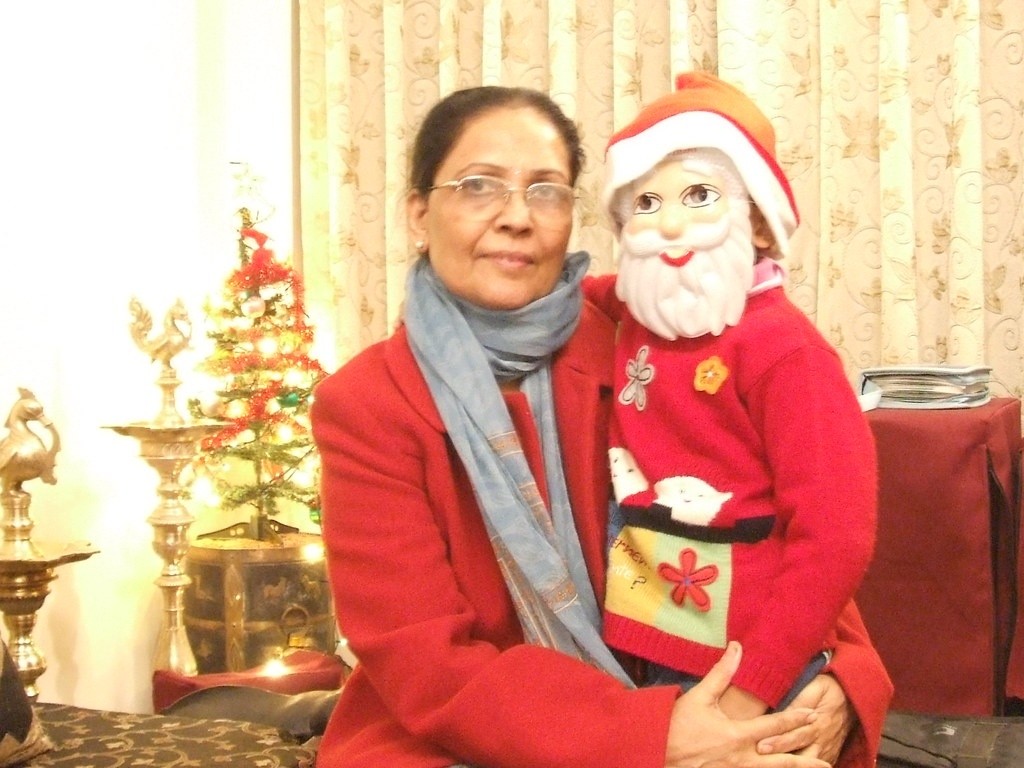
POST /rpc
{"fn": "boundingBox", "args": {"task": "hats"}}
[602,71,801,261]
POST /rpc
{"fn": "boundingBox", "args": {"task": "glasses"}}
[426,175,579,216]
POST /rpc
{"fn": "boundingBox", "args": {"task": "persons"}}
[579,70,880,720]
[311,84,897,768]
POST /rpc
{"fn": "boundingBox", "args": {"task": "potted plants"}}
[184,209,344,677]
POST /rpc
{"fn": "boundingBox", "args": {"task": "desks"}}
[853,397,1024,720]
